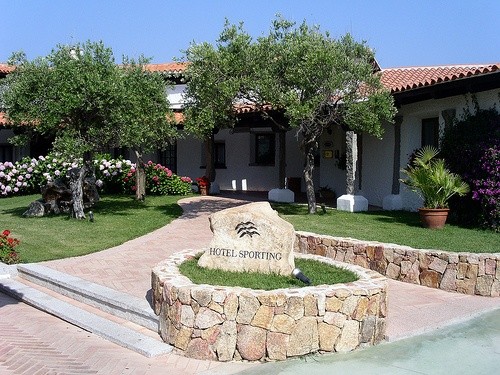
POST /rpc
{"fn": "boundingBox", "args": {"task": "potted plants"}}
[398,144,470,231]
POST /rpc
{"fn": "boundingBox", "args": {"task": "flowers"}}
[196,175,210,187]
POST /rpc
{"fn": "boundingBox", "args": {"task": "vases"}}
[199,186,209,196]
